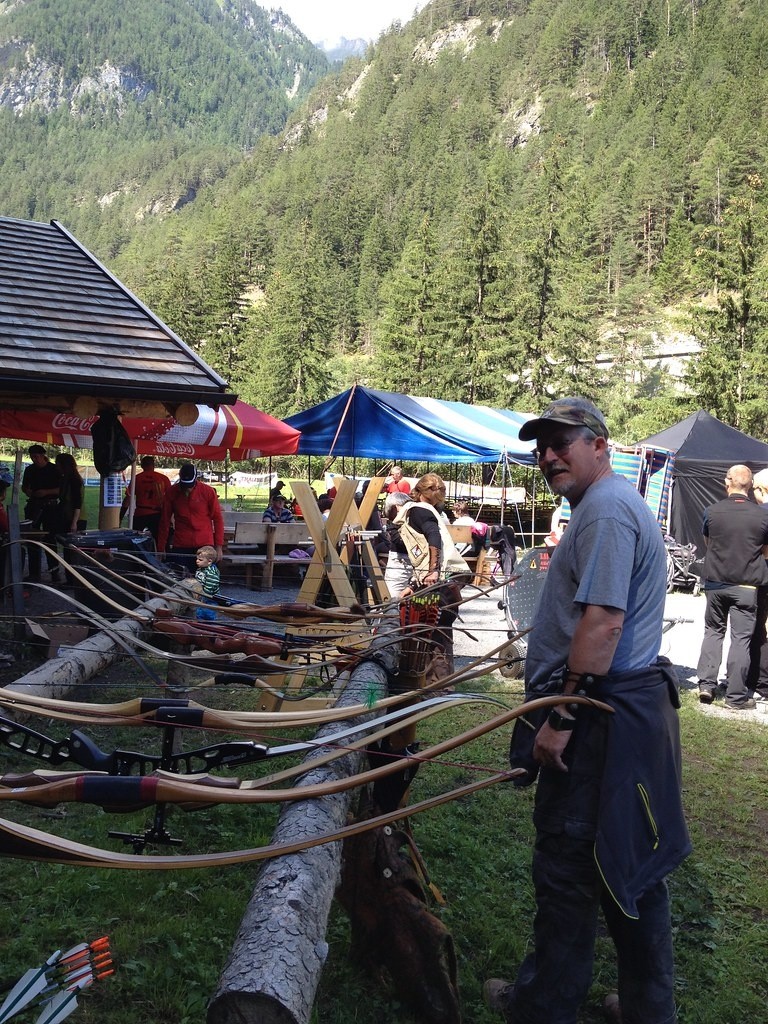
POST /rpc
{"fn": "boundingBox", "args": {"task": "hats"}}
[28,445,46,454]
[179,464,197,486]
[517,397,610,441]
[276,481,286,486]
[272,494,286,500]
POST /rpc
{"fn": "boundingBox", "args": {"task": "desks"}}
[6,531,50,534]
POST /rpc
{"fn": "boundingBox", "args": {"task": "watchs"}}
[548,710,576,731]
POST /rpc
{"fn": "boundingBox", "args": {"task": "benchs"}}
[446,524,514,586]
[222,522,348,592]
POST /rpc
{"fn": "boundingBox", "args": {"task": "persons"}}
[156,464,224,578]
[745,468,768,701]
[452,502,479,557]
[0,479,11,532]
[383,492,471,673]
[310,480,387,557]
[55,453,87,590]
[696,465,768,710]
[385,473,447,598]
[271,481,286,497]
[21,445,61,583]
[257,495,296,553]
[483,401,691,1024]
[119,456,171,531]
[195,546,221,620]
[387,466,410,494]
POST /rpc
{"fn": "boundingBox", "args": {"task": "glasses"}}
[530,440,571,459]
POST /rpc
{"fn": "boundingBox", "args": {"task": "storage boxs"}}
[25,618,89,660]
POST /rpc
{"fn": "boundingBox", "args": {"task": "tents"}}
[268,384,625,549]
[633,409,768,575]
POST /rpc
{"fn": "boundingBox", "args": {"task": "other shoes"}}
[25,575,41,583]
[604,994,622,1024]
[483,977,517,1023]
[726,699,757,710]
[698,689,714,702]
[51,571,60,582]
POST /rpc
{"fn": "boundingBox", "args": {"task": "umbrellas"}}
[0,400,302,527]
